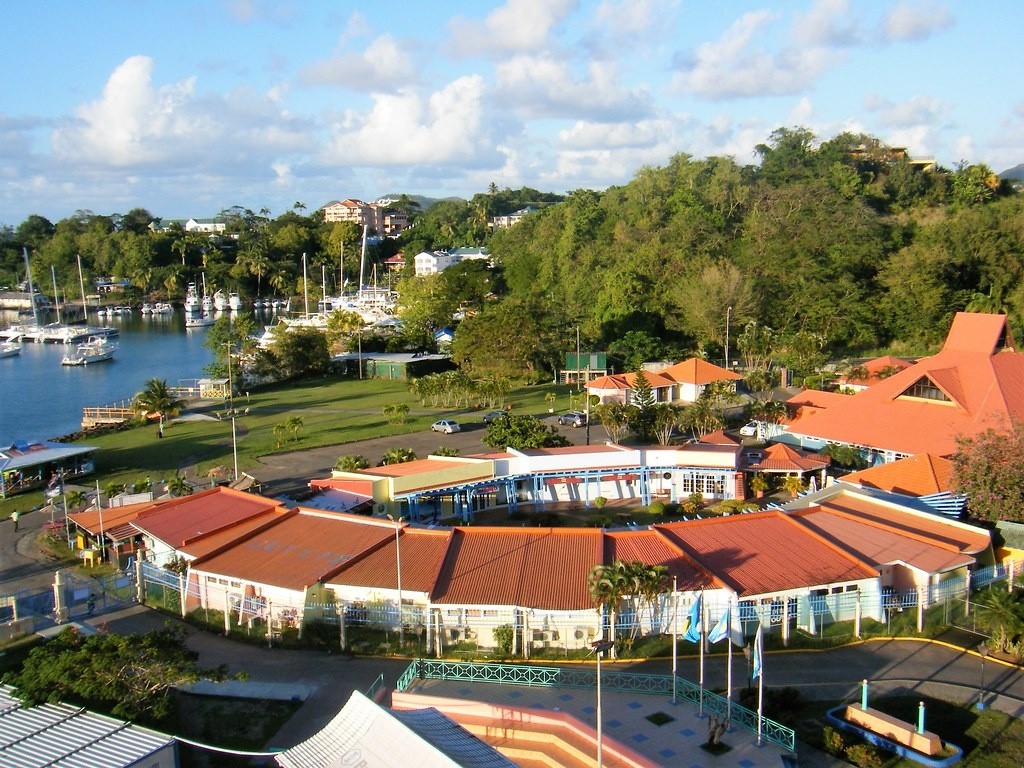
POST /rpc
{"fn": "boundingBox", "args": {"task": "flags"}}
[681,594,700,643]
[708,609,729,644]
[753,623,763,680]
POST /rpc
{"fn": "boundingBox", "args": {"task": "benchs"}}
[616,513,631,520]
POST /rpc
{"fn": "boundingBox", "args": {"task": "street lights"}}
[388,516,407,649]
[592,638,615,767]
[584,365,590,446]
[725,307,732,370]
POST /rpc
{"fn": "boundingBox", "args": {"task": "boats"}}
[0,342,22,358]
[186,316,216,327]
[228,292,242,309]
[202,296,213,311]
[61,336,118,366]
[213,289,227,311]
[0,246,118,344]
[183,282,200,312]
[254,224,403,351]
[98,302,174,316]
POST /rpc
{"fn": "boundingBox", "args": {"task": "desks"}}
[68,539,77,551]
[84,550,101,568]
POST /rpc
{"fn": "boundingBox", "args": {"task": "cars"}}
[483,410,509,424]
[739,419,767,437]
[557,412,586,428]
[431,420,461,435]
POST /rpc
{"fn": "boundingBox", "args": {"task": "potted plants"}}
[749,477,769,500]
[545,392,556,413]
[784,476,804,498]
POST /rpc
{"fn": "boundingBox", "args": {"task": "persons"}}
[11,509,19,533]
[87,593,95,614]
[146,475,150,484]
